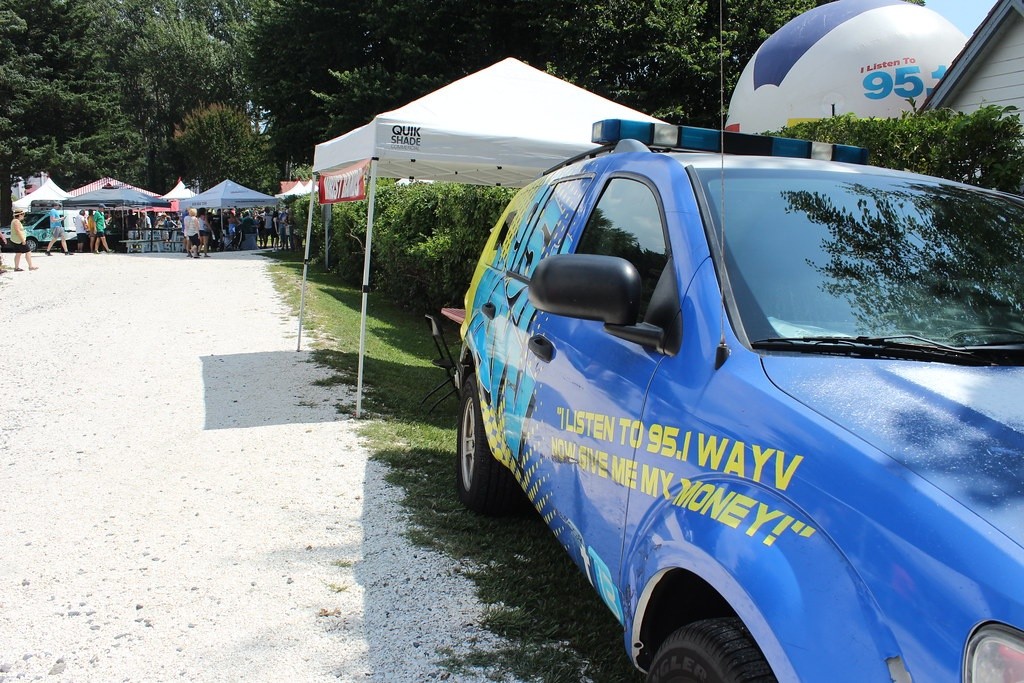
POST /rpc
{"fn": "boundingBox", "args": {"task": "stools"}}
[239,233,259,250]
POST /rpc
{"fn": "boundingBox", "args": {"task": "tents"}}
[11,179,319,251]
[296,56,676,420]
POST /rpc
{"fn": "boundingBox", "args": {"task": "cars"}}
[451,118,1024,682]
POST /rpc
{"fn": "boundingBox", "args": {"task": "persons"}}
[0,202,290,271]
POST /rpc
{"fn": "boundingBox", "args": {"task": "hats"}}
[13,208,25,216]
[98,203,107,208]
[54,201,62,207]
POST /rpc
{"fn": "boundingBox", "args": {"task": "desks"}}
[440,308,466,325]
[129,227,181,253]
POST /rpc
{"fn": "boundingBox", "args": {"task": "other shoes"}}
[45,251,51,256]
[106,249,116,253]
[65,252,73,255]
[29,266,38,271]
[94,249,100,255]
[14,267,23,271]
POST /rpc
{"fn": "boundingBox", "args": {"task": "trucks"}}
[0,200,105,253]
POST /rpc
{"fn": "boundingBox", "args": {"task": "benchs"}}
[118,239,183,252]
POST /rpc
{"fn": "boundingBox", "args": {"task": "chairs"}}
[419,313,461,423]
